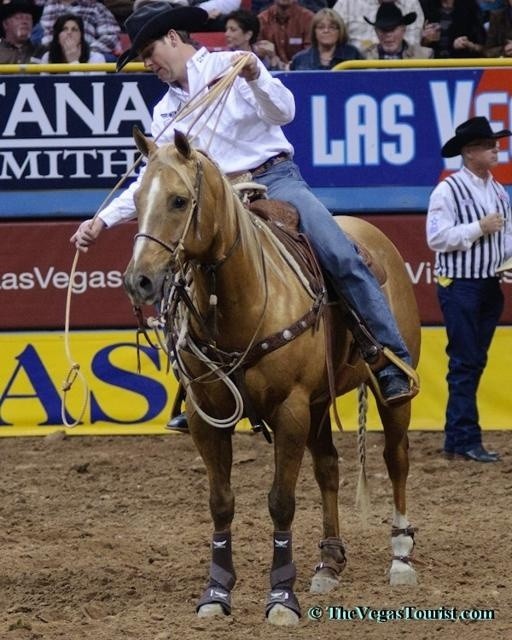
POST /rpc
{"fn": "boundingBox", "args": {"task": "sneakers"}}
[166,409,187,428]
[382,374,409,395]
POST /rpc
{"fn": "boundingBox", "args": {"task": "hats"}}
[0,0,44,26]
[440,115,512,158]
[363,1,417,30]
[115,0,209,73]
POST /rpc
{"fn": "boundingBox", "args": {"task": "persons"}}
[426,117,512,465]
[69,1,412,405]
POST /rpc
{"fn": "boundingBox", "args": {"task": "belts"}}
[249,151,290,178]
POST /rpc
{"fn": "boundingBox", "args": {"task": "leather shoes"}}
[443,440,500,464]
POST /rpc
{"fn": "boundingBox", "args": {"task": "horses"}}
[123,125,422,627]
